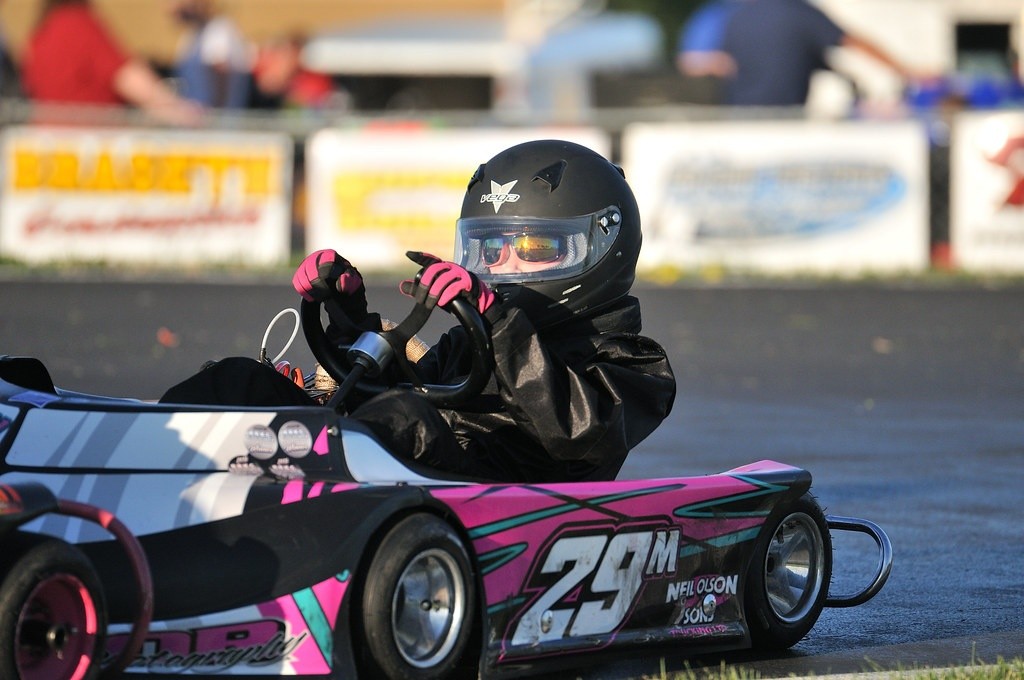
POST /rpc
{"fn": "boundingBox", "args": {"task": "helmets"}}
[453,139,642,325]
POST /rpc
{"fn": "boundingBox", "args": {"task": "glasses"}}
[480,232,568,267]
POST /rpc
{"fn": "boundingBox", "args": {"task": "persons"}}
[158,139,676,482]
[0,1,327,118]
[679,0,934,112]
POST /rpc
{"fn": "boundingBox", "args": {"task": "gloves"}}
[292,249,363,302]
[399,251,495,314]
